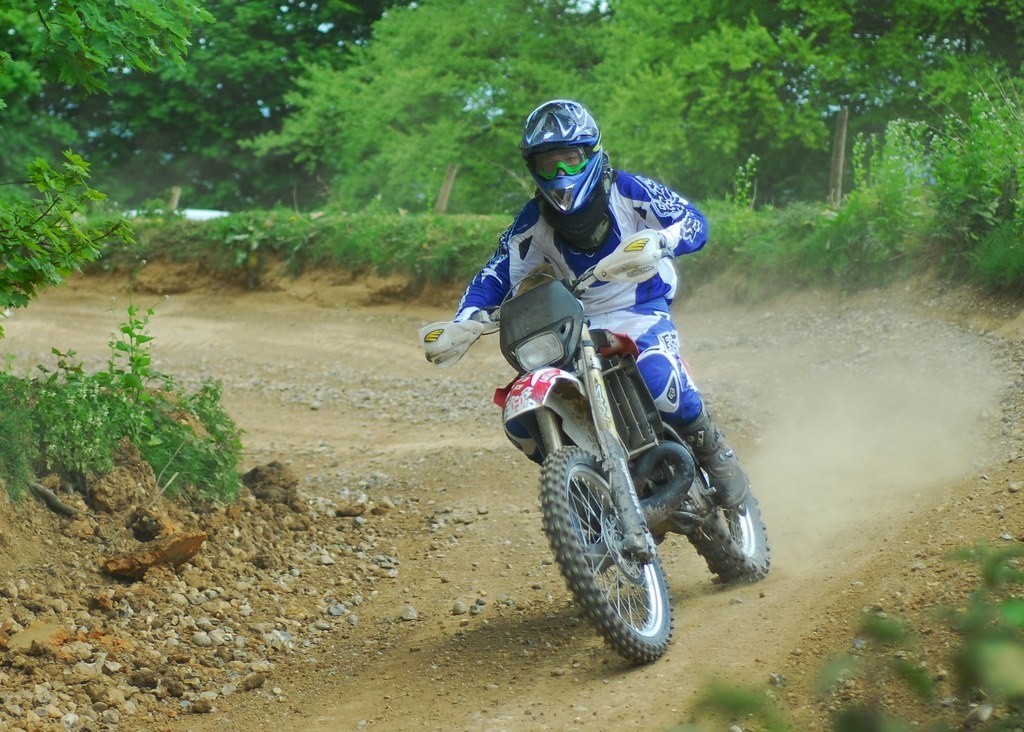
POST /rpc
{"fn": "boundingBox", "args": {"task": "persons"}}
[454,99,749,508]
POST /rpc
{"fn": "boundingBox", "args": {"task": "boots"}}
[681,410,747,508]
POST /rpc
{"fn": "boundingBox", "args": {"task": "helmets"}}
[518,99,604,215]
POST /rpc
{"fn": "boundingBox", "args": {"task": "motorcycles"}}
[425,236,771,663]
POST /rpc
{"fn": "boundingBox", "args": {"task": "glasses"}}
[528,142,589,180]
